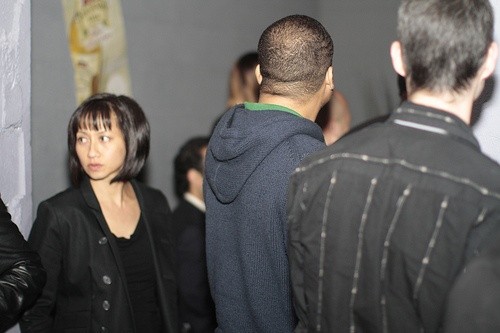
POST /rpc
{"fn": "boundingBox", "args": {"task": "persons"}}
[312,89,351,146]
[227,52,261,105]
[283,1,500,333]
[0,193,47,333]
[203,11,335,333]
[18,92,180,332]
[172,133,218,333]
